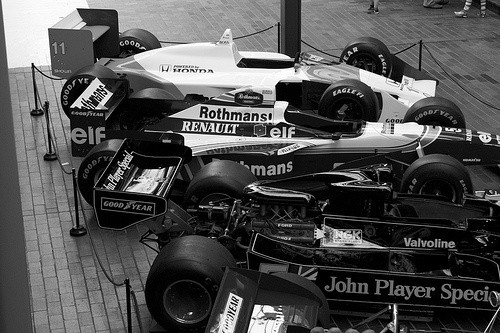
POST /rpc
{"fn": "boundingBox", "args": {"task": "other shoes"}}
[424,3,442,9]
[367,5,379,14]
[454,10,468,18]
[480,12,486,17]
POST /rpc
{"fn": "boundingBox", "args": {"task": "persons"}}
[454,0,487,17]
[423,0,443,8]
[368,0,380,14]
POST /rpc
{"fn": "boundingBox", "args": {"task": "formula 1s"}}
[47,7,500,333]
